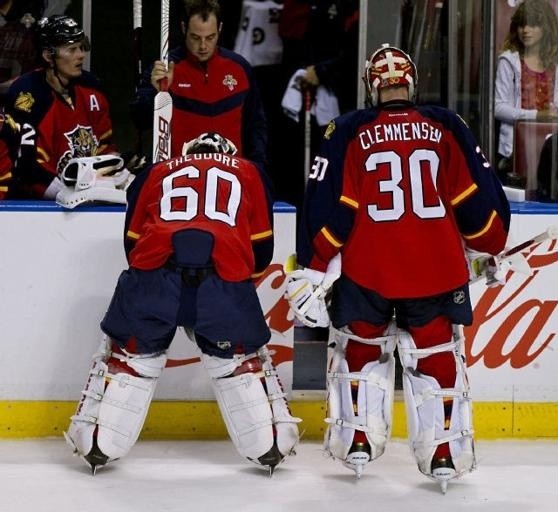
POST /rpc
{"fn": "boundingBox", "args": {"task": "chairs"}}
[512,116,557,200]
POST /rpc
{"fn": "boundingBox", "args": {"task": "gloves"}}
[283,251,343,329]
[61,154,132,189]
[465,242,513,289]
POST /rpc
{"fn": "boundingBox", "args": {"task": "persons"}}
[282,41,511,482]
[1,2,360,210]
[494,0,558,187]
[60,132,304,472]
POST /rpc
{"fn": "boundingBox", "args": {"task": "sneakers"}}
[84,435,110,465]
[258,435,285,465]
[347,441,371,462]
[431,456,456,475]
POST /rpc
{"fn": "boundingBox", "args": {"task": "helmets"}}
[180,131,238,156]
[29,12,85,50]
[365,45,419,102]
